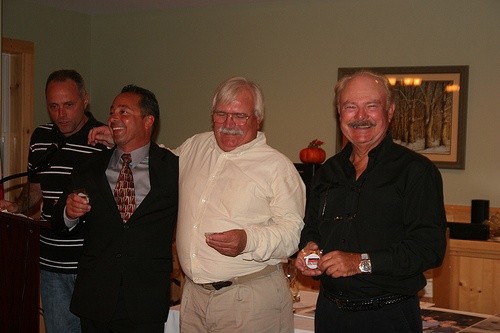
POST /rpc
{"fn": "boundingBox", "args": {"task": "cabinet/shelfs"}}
[446,238,500,315]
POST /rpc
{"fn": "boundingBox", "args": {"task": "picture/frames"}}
[336,64,469,170]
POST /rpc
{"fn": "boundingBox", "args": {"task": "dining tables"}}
[165,291,500,333]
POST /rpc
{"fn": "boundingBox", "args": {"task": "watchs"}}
[359,252,372,272]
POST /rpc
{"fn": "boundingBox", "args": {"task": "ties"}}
[113,153,136,223]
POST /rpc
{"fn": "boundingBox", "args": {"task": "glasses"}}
[212,109,255,126]
[320,179,366,224]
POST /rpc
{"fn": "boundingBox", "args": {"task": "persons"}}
[51,84,181,332]
[26,69,111,333]
[87,76,304,333]
[296,69,447,333]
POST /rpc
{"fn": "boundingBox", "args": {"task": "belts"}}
[324,289,409,311]
[198,263,282,290]
